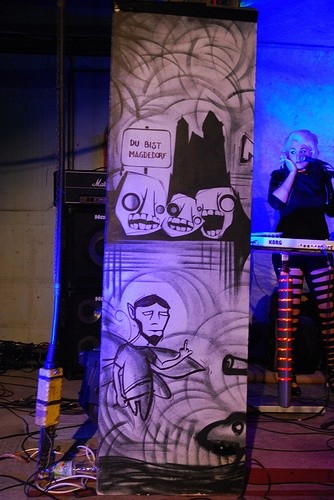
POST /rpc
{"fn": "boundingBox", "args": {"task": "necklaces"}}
[295,163,310,178]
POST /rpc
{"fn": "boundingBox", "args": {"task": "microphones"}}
[300,155,327,165]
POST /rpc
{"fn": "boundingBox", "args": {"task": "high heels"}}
[325,378,334,394]
[290,375,301,395]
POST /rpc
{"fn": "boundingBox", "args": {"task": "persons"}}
[269,129,334,394]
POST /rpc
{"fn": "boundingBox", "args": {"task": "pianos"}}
[250,232,334,254]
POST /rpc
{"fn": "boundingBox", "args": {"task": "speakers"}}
[55,203,108,380]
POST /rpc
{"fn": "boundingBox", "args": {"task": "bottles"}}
[49,460,97,477]
